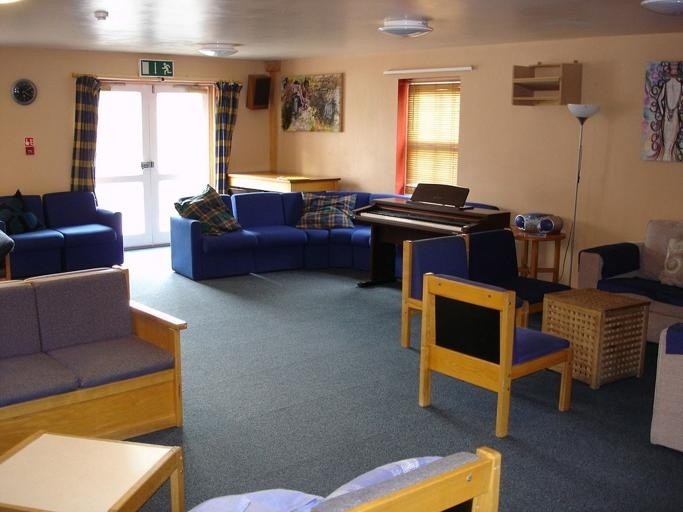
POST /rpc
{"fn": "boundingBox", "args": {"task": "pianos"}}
[350,184,511,289]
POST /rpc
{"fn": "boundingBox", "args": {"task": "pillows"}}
[661,237,682,285]
[174,183,243,236]
[295,191,360,231]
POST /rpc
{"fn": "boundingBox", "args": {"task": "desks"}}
[0,426,185,511]
[223,173,341,194]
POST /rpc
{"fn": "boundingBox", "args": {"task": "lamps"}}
[376,14,433,40]
[640,0,683,17]
[565,102,601,289]
[195,43,241,59]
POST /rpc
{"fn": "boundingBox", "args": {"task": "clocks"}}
[10,79,37,106]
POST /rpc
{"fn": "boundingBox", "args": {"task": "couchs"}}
[168,191,500,293]
[650,322,683,452]
[577,221,682,344]
[1,188,125,282]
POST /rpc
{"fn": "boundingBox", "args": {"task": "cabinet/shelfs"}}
[509,63,582,108]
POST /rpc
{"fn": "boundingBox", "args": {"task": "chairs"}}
[418,272,573,437]
[401,225,569,348]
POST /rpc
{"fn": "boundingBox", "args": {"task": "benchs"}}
[185,442,507,511]
[0,264,189,461]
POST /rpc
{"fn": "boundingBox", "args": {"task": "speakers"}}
[247,75,271,110]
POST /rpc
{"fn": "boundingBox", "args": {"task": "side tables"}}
[541,289,652,390]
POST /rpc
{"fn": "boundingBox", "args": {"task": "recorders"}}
[514,214,562,234]
